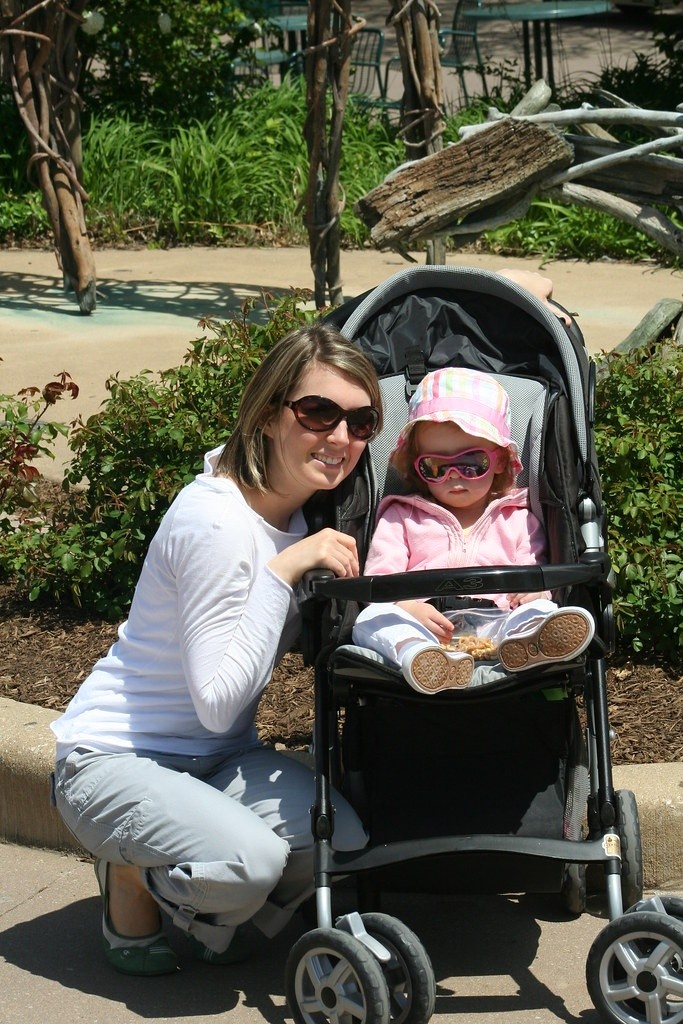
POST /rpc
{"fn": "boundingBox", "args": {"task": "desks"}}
[460,0,615,105]
[267,15,358,85]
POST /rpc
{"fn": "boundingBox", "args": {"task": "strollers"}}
[284,264,682,1024]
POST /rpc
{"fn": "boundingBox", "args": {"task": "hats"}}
[389,367,524,493]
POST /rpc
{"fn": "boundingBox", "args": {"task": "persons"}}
[47,327,384,976]
[352,364,595,694]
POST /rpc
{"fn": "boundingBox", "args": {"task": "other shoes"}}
[401,642,474,695]
[94,857,177,976]
[497,606,595,673]
[184,926,253,965]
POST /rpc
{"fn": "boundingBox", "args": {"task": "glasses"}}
[282,395,379,439]
[413,448,502,485]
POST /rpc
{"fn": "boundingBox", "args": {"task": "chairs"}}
[221,0,491,140]
